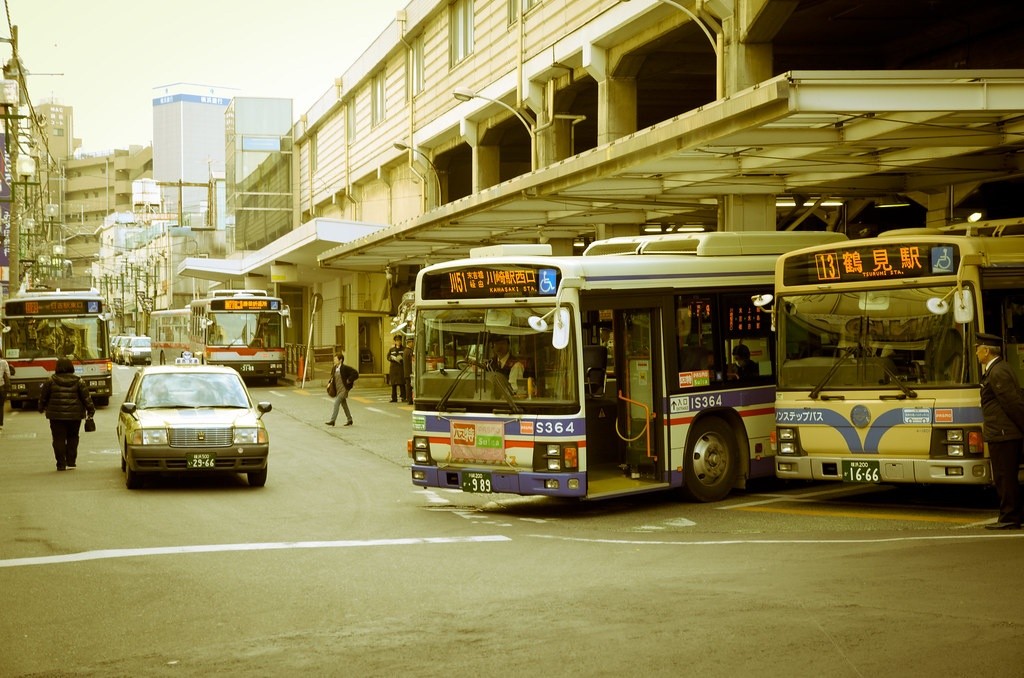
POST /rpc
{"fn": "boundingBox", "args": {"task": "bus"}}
[188,289,293,384]
[752,216,1024,491]
[390,230,852,503]
[0,287,113,408]
[148,308,190,366]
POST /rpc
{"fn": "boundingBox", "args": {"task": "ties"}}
[498,361,502,369]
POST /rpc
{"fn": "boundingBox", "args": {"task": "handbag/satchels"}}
[327,377,336,397]
[85,417,97,432]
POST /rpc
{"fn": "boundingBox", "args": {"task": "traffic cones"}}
[296,356,310,382]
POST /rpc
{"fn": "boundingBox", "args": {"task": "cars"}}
[109,334,152,366]
[116,351,274,490]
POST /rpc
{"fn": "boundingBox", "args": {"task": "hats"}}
[491,334,510,342]
[394,335,402,340]
[406,338,414,342]
[973,333,1003,348]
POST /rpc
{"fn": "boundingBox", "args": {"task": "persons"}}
[927,332,968,383]
[973,333,1024,530]
[325,353,359,426]
[679,331,759,379]
[387,335,406,402]
[485,338,524,390]
[0,349,10,426]
[1008,303,1024,343]
[403,337,414,405]
[862,328,894,357]
[38,357,95,471]
[148,381,174,402]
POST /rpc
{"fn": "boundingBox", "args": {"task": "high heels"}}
[344,421,353,426]
[325,421,335,426]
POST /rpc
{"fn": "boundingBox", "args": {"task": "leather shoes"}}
[986,519,1021,530]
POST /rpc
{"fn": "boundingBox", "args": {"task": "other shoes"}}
[408,400,413,405]
[56,464,65,471]
[402,399,408,402]
[390,398,397,402]
[65,460,76,467]
